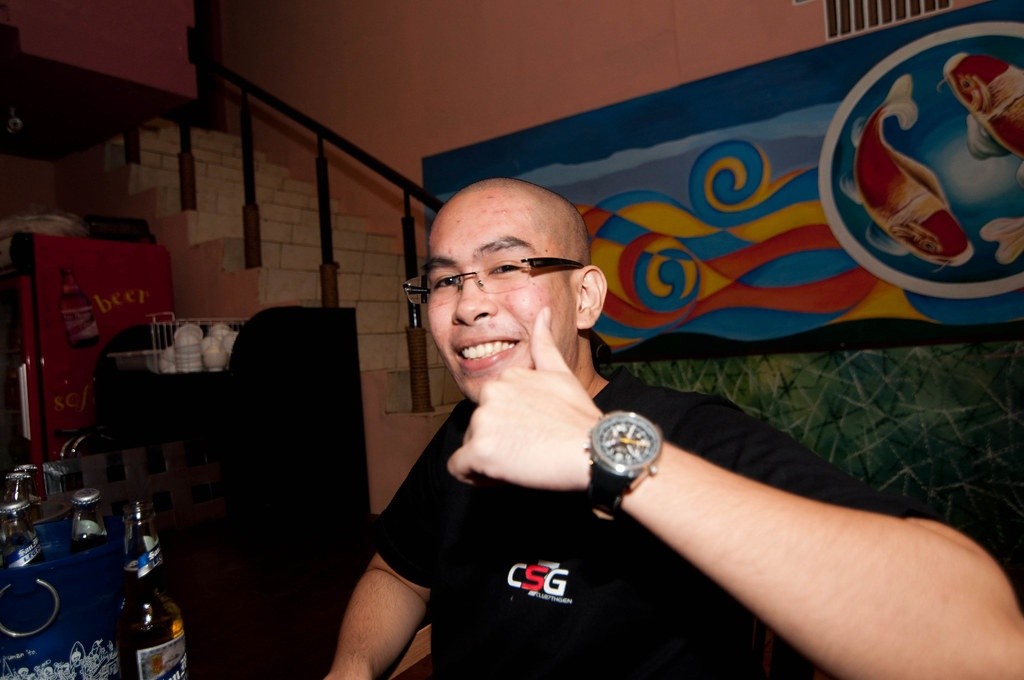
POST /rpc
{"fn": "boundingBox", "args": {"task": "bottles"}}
[10,464,42,520]
[69,487,108,552]
[116,499,189,680]
[0,500,45,569]
[58,267,100,348]
[0,473,31,521]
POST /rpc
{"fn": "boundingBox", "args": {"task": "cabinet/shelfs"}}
[93,304,370,680]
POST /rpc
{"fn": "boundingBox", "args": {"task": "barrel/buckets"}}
[0,517,170,680]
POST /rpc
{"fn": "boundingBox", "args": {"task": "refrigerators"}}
[1,232,176,463]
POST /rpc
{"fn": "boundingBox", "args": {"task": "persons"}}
[323,178,1024,680]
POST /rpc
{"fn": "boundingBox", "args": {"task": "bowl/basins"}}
[159,322,240,372]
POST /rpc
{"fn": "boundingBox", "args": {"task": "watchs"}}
[583,410,663,522]
